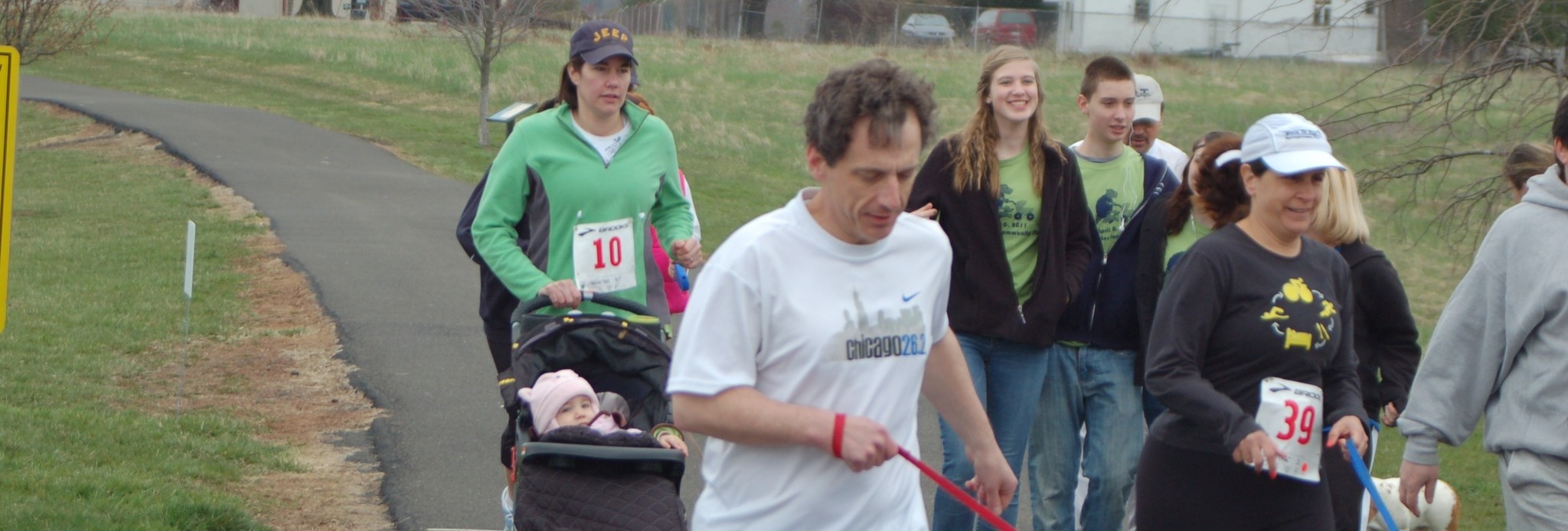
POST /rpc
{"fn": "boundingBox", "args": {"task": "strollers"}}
[494,289,691,531]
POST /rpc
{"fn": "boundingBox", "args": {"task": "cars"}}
[899,12,957,46]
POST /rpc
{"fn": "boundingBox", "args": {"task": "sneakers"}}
[501,485,517,531]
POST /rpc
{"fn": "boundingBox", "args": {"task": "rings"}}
[691,258,693,262]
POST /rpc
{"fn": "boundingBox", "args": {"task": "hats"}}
[518,369,601,436]
[1240,114,1348,175]
[1132,71,1164,123]
[569,19,639,65]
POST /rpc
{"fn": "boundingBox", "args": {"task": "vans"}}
[969,7,1038,48]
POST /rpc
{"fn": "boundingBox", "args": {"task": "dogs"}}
[1369,474,1461,531]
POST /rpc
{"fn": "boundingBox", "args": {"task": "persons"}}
[453,25,1568,531]
